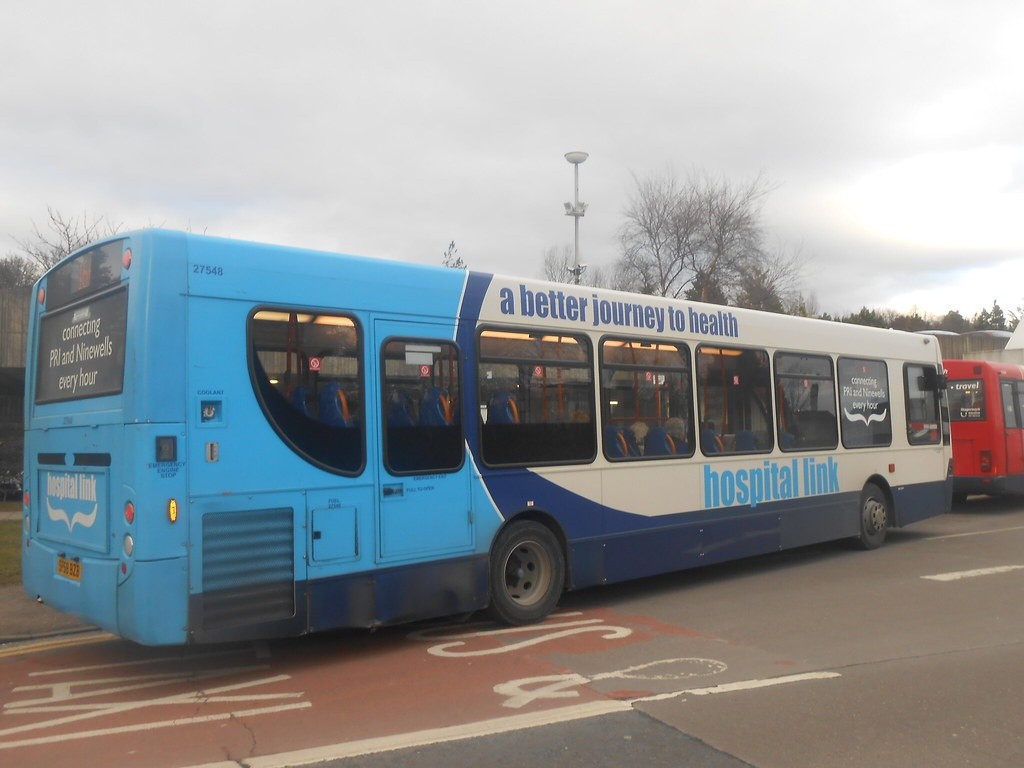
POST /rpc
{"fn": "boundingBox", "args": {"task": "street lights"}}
[564,151,589,285]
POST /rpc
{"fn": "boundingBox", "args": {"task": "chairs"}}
[607,425,796,457]
[274,377,520,428]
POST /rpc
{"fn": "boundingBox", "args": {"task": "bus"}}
[911,359,1024,494]
[22,228,953,648]
